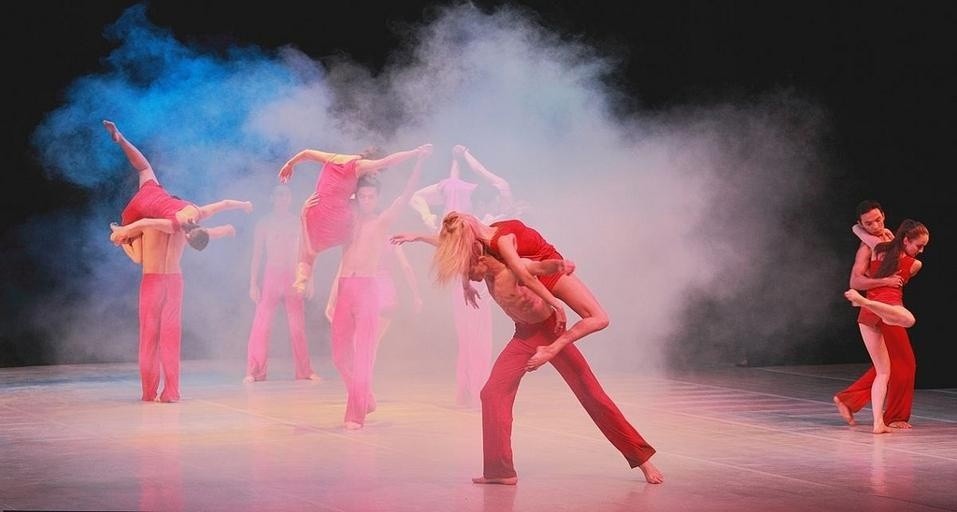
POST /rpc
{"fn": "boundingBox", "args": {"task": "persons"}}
[101,119,252,251]
[111,195,234,403]
[277,139,513,430]
[845,220,931,432]
[434,208,609,372]
[389,230,665,485]
[833,203,917,430]
[243,186,321,384]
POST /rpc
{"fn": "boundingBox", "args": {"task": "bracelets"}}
[464,285,471,291]
